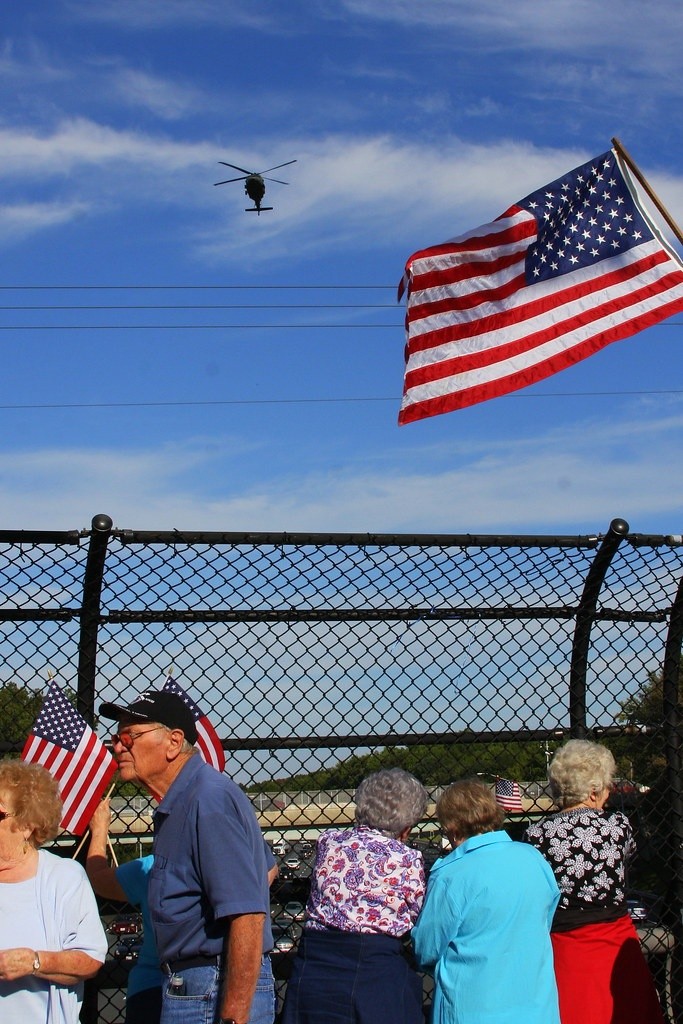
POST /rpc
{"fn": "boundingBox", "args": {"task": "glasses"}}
[0,811,14,822]
[111,726,164,749]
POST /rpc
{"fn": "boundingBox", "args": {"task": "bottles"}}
[168,975,185,996]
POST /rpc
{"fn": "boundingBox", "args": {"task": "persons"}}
[0,692,668,1024]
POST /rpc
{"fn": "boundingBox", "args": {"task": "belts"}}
[170,952,270,973]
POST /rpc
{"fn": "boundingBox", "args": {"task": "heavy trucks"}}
[299,844,313,859]
[270,837,289,855]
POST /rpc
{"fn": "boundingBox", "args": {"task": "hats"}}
[98,690,198,746]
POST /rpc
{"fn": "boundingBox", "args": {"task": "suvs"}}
[268,919,298,954]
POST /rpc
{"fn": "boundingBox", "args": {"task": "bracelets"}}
[219,1016,239,1024]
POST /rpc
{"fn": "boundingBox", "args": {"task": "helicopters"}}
[212,160,298,214]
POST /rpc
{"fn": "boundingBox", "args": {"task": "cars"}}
[523,786,553,799]
[281,901,304,921]
[110,804,148,818]
[276,866,294,882]
[253,800,285,812]
[429,842,438,848]
[285,859,299,870]
[410,842,419,849]
[109,913,142,936]
[114,937,144,959]
[611,777,651,796]
[624,900,648,921]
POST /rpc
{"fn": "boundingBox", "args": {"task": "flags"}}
[493,773,523,813]
[391,147,683,428]
[159,673,226,777]
[20,679,120,838]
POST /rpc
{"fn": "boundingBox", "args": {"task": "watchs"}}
[31,948,41,975]
